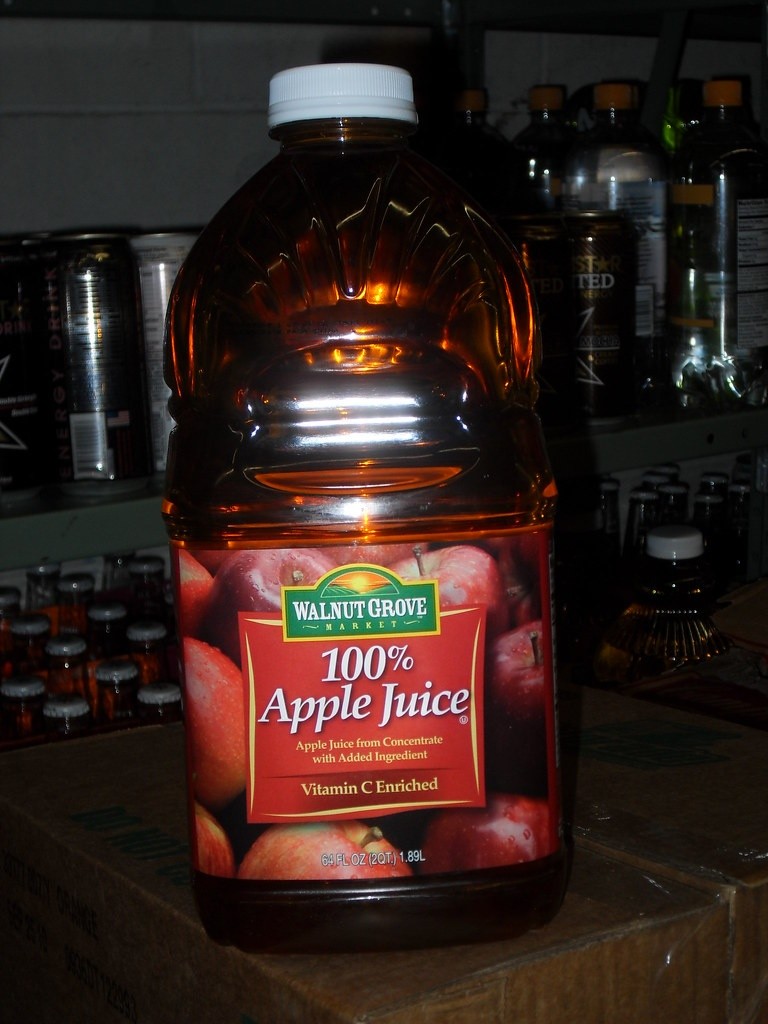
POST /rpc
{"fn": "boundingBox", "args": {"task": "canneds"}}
[502,208,637,377]
[0,230,200,507]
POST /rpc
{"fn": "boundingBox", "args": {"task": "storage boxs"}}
[0,674,767,1024]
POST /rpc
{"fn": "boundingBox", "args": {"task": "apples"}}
[178,538,557,879]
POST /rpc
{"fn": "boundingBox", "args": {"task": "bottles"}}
[447,77,768,721]
[1,553,181,751]
[162,62,571,953]
